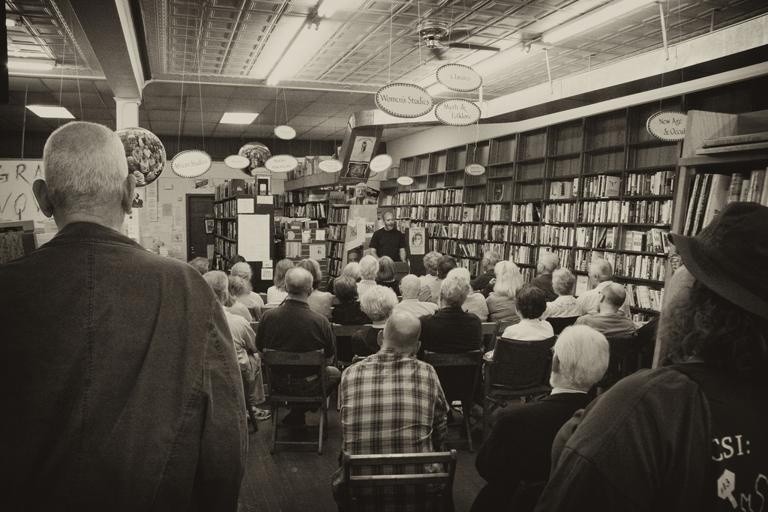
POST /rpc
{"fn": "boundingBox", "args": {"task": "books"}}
[213,157,348,276]
[382,170,766,329]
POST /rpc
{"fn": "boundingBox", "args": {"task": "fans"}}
[394,24,502,63]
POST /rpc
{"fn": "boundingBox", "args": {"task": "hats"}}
[668,202,762,320]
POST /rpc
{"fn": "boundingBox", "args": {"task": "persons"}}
[188,212,639,425]
[0,120,250,512]
[470,324,611,512]
[534,202,768,512]
[337,310,452,511]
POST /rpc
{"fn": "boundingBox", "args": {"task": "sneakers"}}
[246,406,272,420]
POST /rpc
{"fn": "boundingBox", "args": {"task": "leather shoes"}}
[282,412,305,424]
